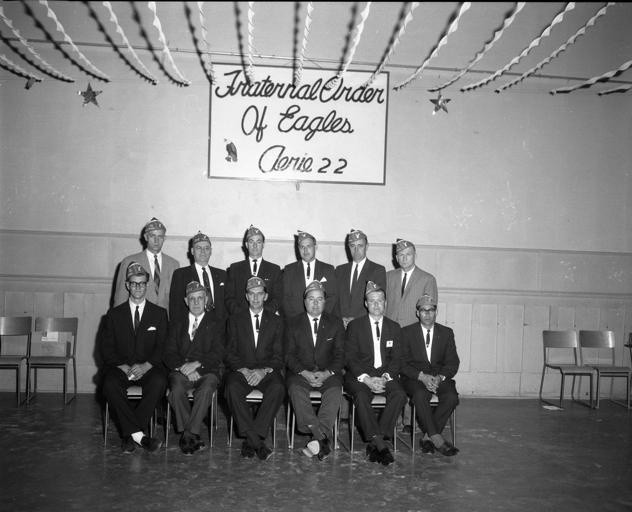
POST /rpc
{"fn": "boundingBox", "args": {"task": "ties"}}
[255,310,261,337]
[251,259,259,277]
[425,328,433,348]
[373,320,383,340]
[352,263,361,297]
[153,254,161,296]
[133,305,140,336]
[192,317,199,339]
[312,315,319,338]
[306,262,313,282]
[400,272,408,297]
[201,266,211,292]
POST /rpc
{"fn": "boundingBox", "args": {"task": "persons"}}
[170,232,226,313]
[386,238,438,327]
[284,279,346,460]
[400,295,461,456]
[227,223,283,314]
[345,282,402,465]
[168,280,221,457]
[94,262,168,455]
[112,217,179,309]
[282,231,335,312]
[335,228,386,328]
[223,277,284,461]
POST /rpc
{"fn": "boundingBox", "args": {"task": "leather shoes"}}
[133,434,162,454]
[434,442,460,457]
[178,436,194,456]
[318,443,332,461]
[190,434,205,450]
[365,444,390,466]
[419,438,435,454]
[253,443,274,462]
[377,444,395,463]
[241,439,256,460]
[302,439,322,459]
[121,437,138,454]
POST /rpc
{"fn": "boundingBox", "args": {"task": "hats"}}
[346,229,366,244]
[189,230,212,249]
[395,238,413,253]
[365,280,381,295]
[245,224,263,241]
[126,262,146,281]
[246,277,268,291]
[416,293,437,308]
[185,280,208,297]
[303,280,327,300]
[143,216,167,233]
[296,229,317,246]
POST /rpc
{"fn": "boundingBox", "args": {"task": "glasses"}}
[128,281,147,288]
[147,233,166,240]
[419,307,437,314]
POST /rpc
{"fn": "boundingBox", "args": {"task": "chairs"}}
[286,387,342,451]
[579,330,631,411]
[338,386,398,455]
[103,382,156,448]
[227,387,277,450]
[396,392,458,456]
[0,315,33,409]
[539,329,594,410]
[26,317,79,407]
[162,389,219,450]
